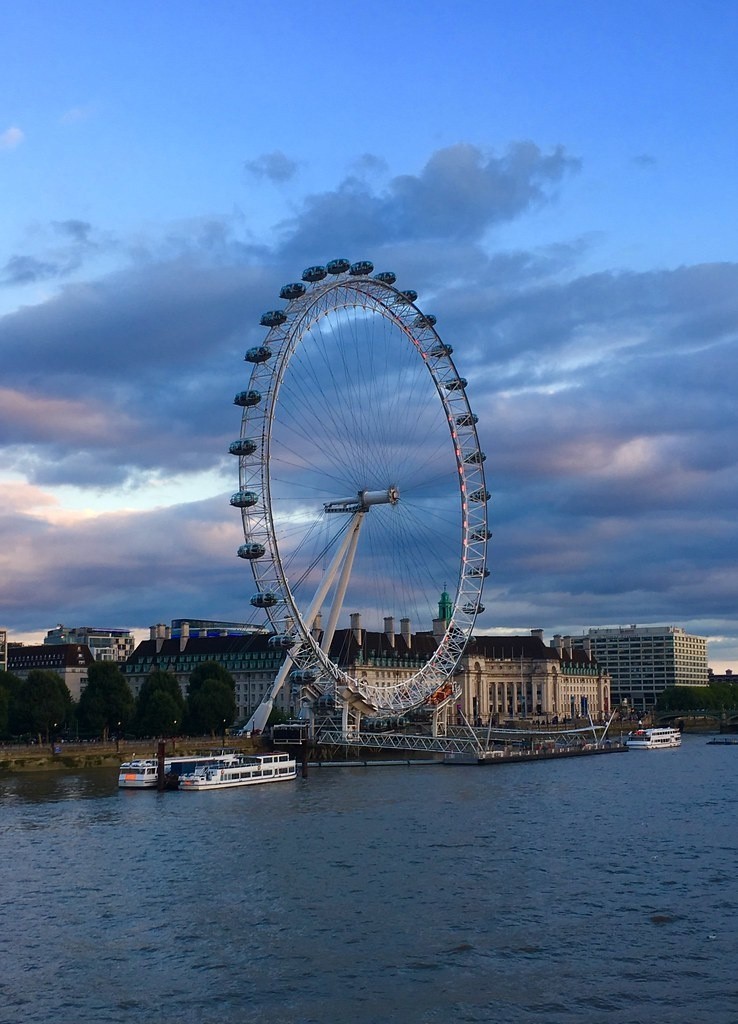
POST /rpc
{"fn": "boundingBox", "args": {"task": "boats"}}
[626,727,682,750]
[174,753,297,791]
[117,748,247,788]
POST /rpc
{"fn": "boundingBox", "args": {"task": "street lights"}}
[52,723,57,755]
[116,722,121,751]
[222,718,226,748]
[173,720,177,753]
[253,717,255,736]
